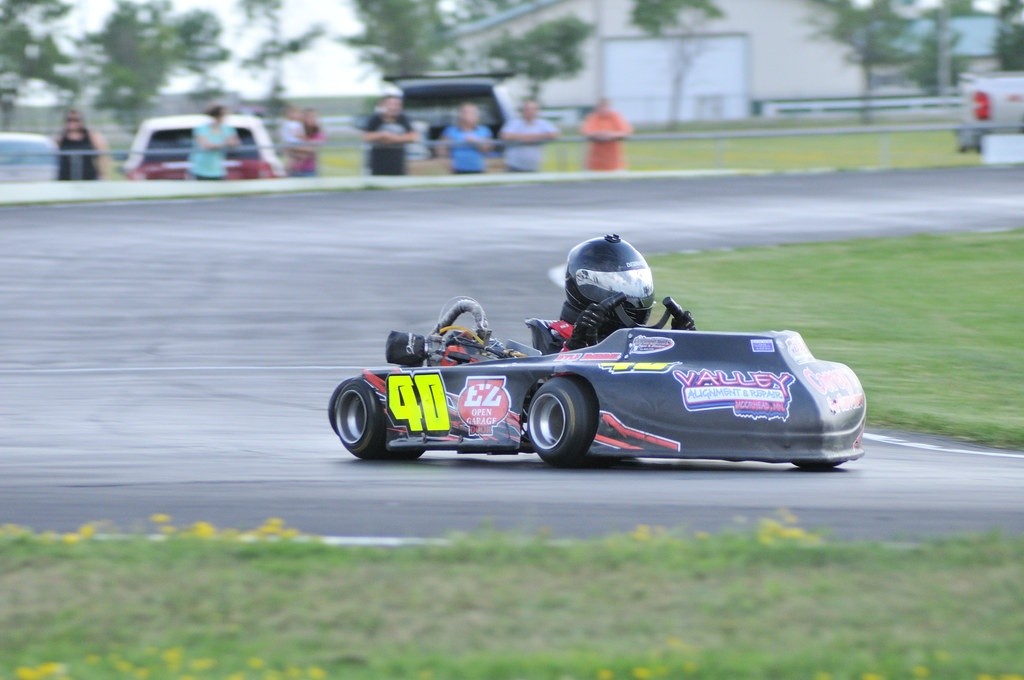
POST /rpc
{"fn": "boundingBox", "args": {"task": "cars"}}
[362,69,534,175]
[955,71,1024,152]
[1,131,62,182]
[119,113,287,181]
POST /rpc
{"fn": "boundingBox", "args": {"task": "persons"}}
[188,102,244,180]
[547,233,698,355]
[47,108,112,180]
[579,97,633,171]
[496,97,562,173]
[356,93,420,177]
[277,103,327,179]
[436,104,494,176]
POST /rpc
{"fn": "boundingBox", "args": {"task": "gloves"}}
[671,311,696,331]
[565,302,609,351]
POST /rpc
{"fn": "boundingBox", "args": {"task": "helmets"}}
[565,234,654,327]
